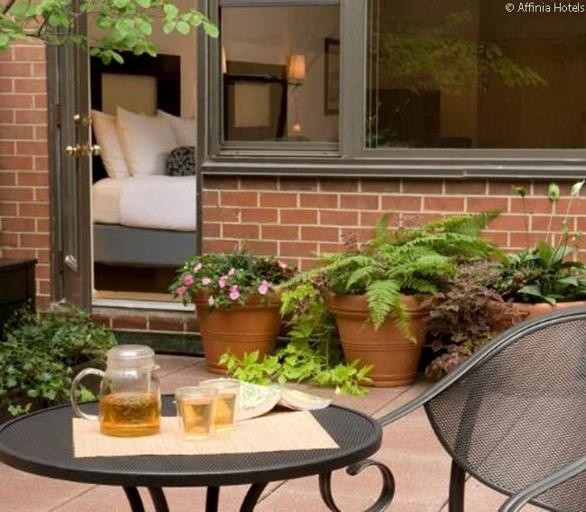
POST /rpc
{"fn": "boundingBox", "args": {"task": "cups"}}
[199,377,241,432]
[173,385,217,441]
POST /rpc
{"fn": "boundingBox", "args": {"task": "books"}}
[197,377,334,423]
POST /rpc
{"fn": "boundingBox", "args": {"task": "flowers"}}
[166,242,292,315]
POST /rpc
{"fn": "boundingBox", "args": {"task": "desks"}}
[0,393,383,512]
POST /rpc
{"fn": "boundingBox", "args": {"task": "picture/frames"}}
[323,37,341,115]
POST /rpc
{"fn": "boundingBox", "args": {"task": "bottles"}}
[69,343,162,438]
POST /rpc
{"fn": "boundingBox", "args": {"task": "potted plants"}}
[0,294,119,419]
[481,175,586,399]
[281,208,513,389]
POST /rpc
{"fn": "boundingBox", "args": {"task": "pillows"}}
[90,109,131,179]
[154,109,197,148]
[115,106,180,179]
[165,147,196,177]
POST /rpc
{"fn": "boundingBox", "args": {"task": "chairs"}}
[318,304,586,512]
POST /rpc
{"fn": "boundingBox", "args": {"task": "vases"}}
[194,290,283,376]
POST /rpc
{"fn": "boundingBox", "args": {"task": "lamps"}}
[287,52,307,87]
[220,43,228,75]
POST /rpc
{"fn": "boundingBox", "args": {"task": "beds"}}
[90,49,197,269]
